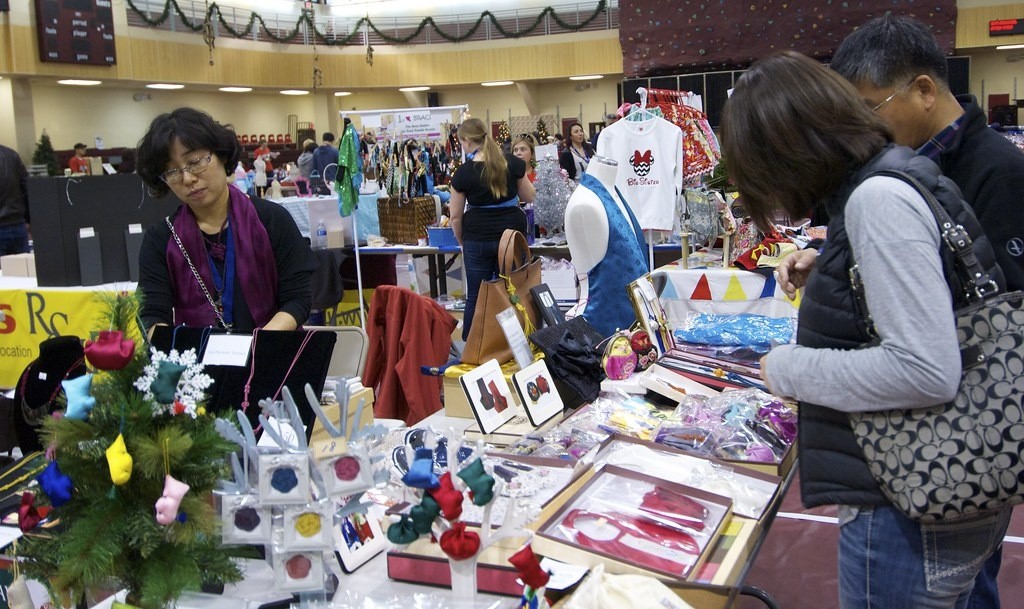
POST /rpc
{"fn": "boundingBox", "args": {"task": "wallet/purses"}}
[599,318,658,380]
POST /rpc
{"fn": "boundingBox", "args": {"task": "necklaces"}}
[201,229,227,315]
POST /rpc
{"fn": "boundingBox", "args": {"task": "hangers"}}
[621,88,708,120]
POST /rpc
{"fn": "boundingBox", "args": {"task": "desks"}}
[355,243,701,300]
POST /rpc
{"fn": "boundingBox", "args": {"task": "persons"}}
[67,143,90,178]
[235,161,248,193]
[254,155,267,186]
[236,118,596,345]
[566,155,651,346]
[0,143,31,256]
[725,51,1008,609]
[828,15,1024,294]
[133,107,315,331]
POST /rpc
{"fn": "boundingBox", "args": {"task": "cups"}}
[65,168,70,176]
[326,229,345,248]
[367,234,385,246]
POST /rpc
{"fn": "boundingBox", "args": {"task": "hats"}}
[303,138,315,150]
[75,143,87,149]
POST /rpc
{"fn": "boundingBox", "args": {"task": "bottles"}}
[318,219,326,247]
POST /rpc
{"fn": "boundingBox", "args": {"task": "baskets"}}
[375,191,442,245]
[427,221,458,247]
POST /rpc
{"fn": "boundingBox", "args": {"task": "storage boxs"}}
[443,376,520,419]
[308,388,376,447]
[541,268,580,306]
[465,407,565,443]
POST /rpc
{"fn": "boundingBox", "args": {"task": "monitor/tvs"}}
[35,0,116,66]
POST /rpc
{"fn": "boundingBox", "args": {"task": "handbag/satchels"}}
[528,315,607,410]
[459,228,544,366]
[846,169,1024,521]
[311,170,331,196]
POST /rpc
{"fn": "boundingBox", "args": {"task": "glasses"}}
[158,150,213,184]
[516,133,534,142]
